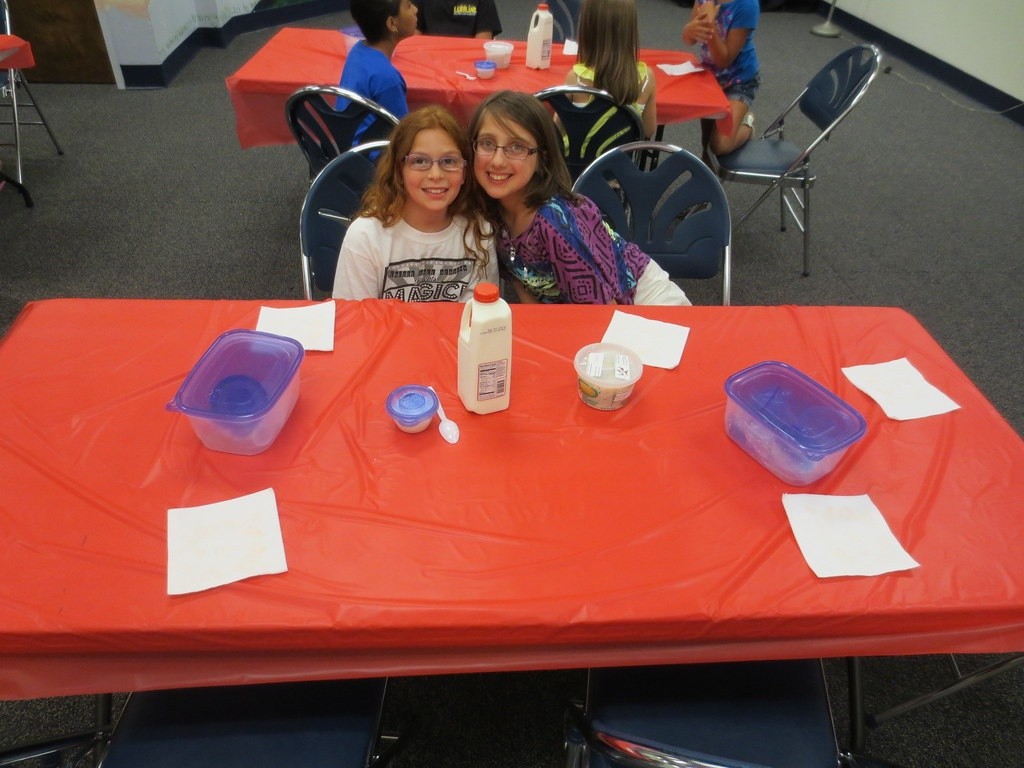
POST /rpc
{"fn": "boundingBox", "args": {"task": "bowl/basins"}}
[385,386,438,433]
[337,25,394,60]
[483,42,514,69]
[724,358,866,486]
[474,60,496,80]
[167,330,305,455]
[573,342,644,411]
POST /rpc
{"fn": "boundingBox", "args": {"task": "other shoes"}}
[743,113,754,128]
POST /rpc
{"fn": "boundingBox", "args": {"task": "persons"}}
[468,90,692,305]
[552,0,656,188]
[409,0,503,39]
[332,105,499,304]
[675,0,761,225]
[334,0,418,161]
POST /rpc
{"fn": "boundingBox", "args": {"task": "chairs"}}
[68,684,419,768]
[706,42,881,276]
[564,659,856,767]
[284,82,734,309]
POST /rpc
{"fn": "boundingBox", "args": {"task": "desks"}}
[2,300,1023,768]
[229,27,733,167]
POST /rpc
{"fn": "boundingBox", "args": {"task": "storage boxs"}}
[473,62,497,81]
[167,328,304,456]
[482,41,513,70]
[574,342,643,411]
[723,361,866,486]
[386,384,439,434]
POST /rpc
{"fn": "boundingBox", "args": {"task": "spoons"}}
[456,71,476,81]
[427,386,460,443]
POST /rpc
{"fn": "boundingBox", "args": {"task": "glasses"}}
[405,154,467,171]
[471,138,547,160]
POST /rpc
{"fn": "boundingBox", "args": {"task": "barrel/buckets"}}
[526,4,553,69]
[457,283,513,413]
